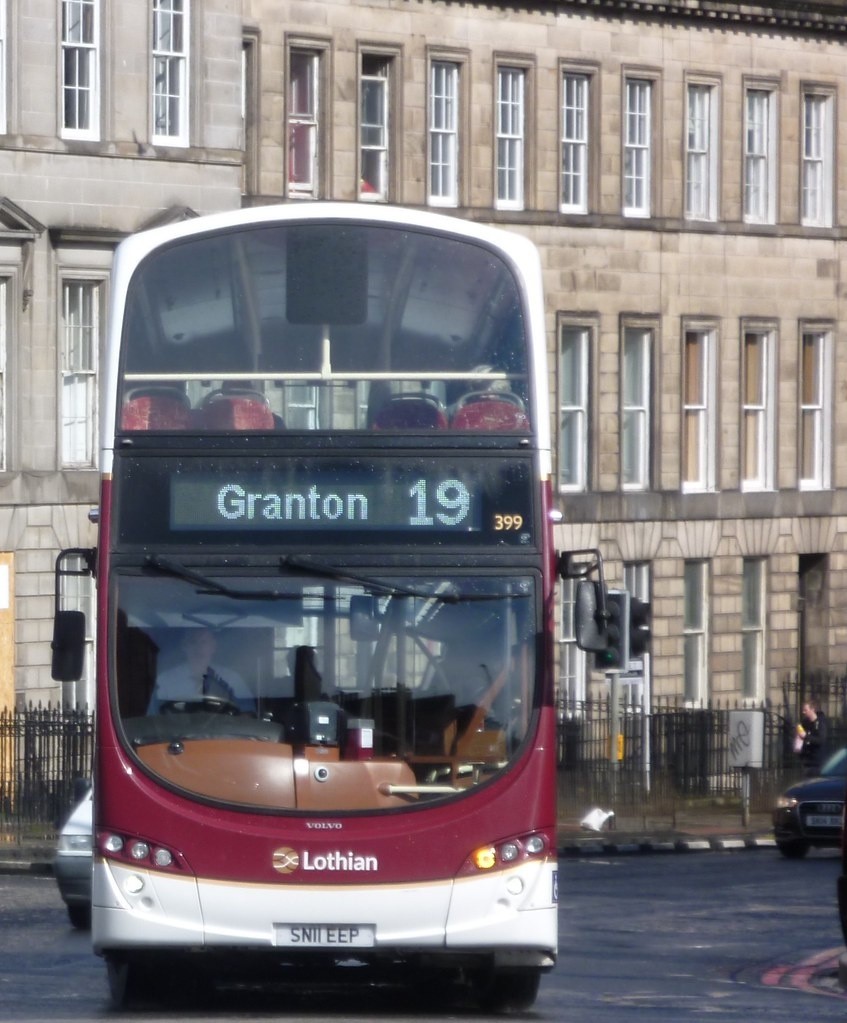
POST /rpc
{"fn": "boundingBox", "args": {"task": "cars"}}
[771,747,847,859]
[57,786,94,930]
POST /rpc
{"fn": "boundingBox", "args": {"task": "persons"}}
[796,701,828,776]
[148,628,259,720]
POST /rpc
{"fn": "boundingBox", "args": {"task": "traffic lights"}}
[595,594,625,670]
[630,595,650,668]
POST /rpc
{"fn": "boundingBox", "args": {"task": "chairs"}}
[122,385,274,429]
[376,390,531,432]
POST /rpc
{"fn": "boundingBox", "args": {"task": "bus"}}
[50,200,611,1011]
